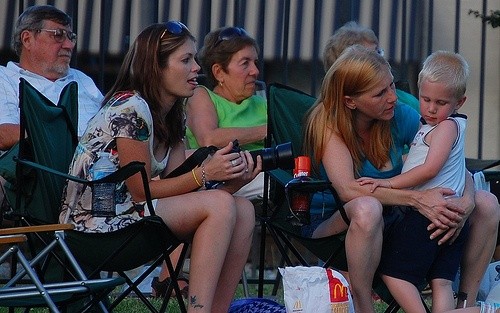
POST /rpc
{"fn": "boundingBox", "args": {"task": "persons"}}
[300,45,499,313]
[59,21,262,313]
[322,21,422,117]
[356,50,470,313]
[0,6,189,300]
[182,27,269,200]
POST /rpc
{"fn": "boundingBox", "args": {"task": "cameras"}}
[208,139,296,172]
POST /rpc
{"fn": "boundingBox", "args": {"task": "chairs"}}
[0,74,500,313]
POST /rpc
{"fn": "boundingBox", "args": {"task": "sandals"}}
[148,276,189,299]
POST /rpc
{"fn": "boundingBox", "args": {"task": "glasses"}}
[161,20,190,41]
[214,27,249,47]
[37,28,77,43]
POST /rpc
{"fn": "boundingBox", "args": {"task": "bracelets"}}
[191,164,206,188]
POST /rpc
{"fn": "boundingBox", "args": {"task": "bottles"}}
[93,152,115,217]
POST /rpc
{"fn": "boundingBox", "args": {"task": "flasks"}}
[292,156,311,225]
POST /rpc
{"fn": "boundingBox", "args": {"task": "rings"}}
[230,159,238,167]
[245,168,248,173]
